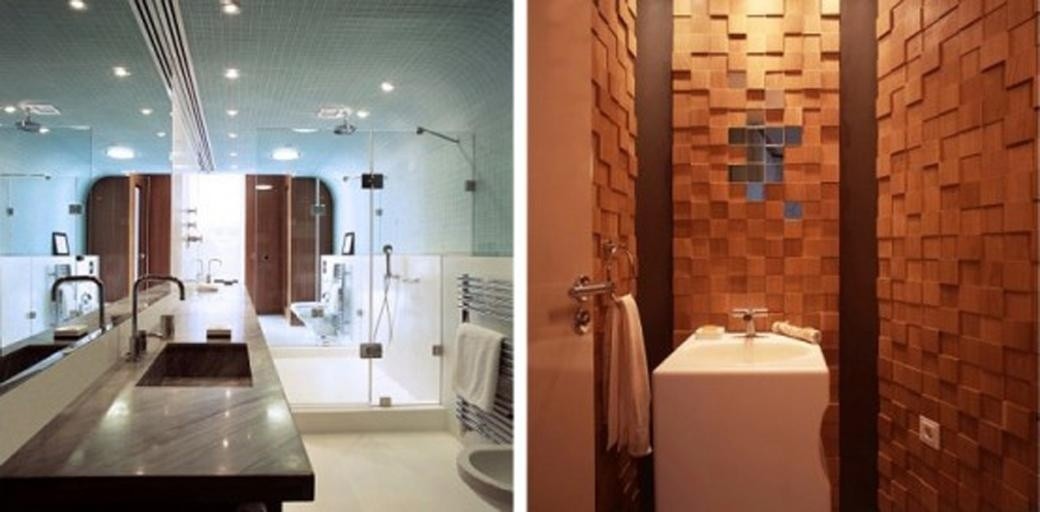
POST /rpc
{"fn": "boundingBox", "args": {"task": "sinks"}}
[686,338,808,367]
[0,344,68,383]
[457,444,513,512]
[289,301,340,338]
[136,340,254,388]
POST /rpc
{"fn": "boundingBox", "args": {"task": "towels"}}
[601,294,653,457]
[451,321,510,414]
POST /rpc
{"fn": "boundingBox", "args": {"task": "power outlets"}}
[919,415,940,451]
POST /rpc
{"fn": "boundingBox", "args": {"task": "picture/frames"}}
[342,232,354,254]
[52,232,69,255]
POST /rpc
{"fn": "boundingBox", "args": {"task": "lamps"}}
[333,111,357,135]
[15,107,49,134]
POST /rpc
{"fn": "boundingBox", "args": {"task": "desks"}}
[0,284,173,393]
[1,283,315,512]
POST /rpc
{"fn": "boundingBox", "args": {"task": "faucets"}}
[51,274,105,326]
[124,275,184,360]
[728,306,768,337]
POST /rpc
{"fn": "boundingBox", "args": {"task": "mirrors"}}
[0,1,174,392]
